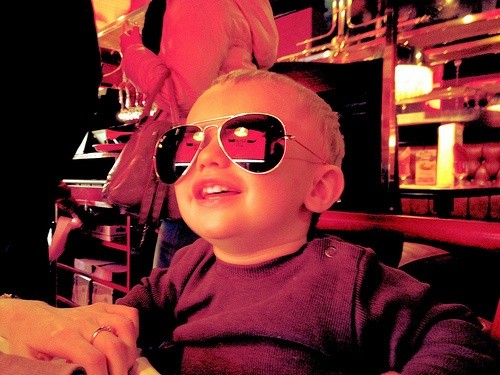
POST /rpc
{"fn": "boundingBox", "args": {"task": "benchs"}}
[398,144,500,219]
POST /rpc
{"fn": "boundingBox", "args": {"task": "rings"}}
[89,324,114,345]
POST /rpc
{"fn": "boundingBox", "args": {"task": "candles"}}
[438,123,455,190]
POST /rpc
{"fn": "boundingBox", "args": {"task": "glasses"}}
[154,113,329,185]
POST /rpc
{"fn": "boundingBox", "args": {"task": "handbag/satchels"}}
[102,70,180,224]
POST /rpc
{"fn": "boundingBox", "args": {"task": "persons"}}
[0,292,141,375]
[120,0,278,270]
[0,0,102,307]
[115,69,495,375]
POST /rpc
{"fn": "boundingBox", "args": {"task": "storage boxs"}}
[70,257,127,304]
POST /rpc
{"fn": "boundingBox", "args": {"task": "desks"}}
[399,183,499,220]
